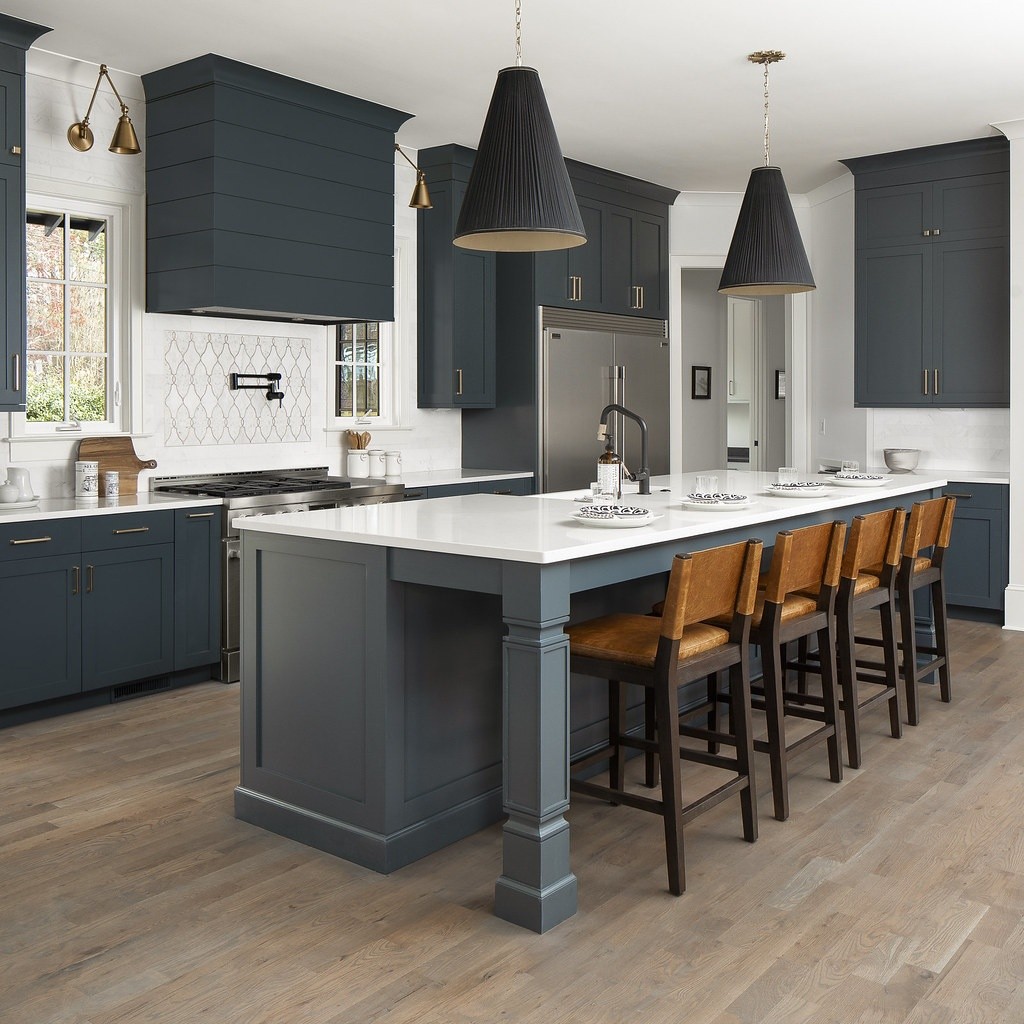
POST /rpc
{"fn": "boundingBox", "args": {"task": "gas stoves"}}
[149,463,404,508]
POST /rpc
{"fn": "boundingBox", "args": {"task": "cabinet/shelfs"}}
[536,155,680,319]
[838,135,1010,408]
[727,297,754,403]
[404,478,532,501]
[0,11,54,411]
[415,143,497,407]
[829,466,1010,610]
[0,505,221,711]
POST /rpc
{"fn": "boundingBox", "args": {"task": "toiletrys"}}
[596,433,622,500]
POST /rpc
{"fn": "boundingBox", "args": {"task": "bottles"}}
[369,451,387,477]
[7,467,33,502]
[385,451,403,475]
[346,450,369,478]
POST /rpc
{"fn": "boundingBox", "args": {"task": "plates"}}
[825,471,893,487]
[565,506,665,527]
[0,500,39,509]
[766,483,827,498]
[680,493,759,511]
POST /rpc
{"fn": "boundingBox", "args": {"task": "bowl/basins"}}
[0,481,18,502]
[882,448,921,471]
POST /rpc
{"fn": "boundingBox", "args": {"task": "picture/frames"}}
[692,366,712,399]
[775,369,785,399]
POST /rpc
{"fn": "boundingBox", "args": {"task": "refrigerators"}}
[536,306,671,495]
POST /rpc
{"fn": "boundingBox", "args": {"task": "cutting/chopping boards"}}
[77,436,157,496]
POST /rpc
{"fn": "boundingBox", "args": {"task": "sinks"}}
[524,488,667,501]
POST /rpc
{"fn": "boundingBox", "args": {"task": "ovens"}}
[221,494,406,687]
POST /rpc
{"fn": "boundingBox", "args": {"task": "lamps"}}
[69,64,142,155]
[394,144,433,208]
[717,50,816,295]
[451,0,588,252]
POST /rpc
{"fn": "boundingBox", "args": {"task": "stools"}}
[569,497,956,895]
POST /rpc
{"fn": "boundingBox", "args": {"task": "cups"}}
[695,475,717,492]
[840,461,859,472]
[589,480,618,505]
[104,471,119,496]
[778,468,798,483]
[75,461,98,496]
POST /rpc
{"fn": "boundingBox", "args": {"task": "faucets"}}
[600,403,653,496]
